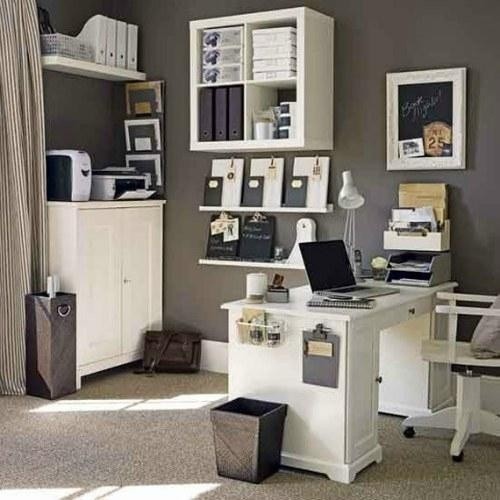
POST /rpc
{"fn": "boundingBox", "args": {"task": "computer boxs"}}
[46,148,93,202]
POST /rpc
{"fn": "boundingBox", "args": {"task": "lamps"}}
[337,169,367,270]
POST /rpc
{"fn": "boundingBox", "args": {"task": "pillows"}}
[469,288,499,360]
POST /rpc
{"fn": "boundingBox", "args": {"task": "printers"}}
[92,167,157,200]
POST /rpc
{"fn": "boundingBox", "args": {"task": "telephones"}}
[350,250,375,278]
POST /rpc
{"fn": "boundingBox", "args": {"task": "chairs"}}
[402,291,500,462]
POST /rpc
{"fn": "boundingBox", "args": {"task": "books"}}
[306,289,376,309]
[123,80,163,116]
[124,153,164,187]
[198,153,332,263]
[124,119,163,152]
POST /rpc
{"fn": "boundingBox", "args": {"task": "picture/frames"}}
[385,66,467,173]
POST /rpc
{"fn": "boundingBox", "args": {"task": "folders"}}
[198,86,243,142]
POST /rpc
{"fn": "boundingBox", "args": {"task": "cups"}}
[246,273,268,303]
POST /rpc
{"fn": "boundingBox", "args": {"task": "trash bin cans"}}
[210,397,288,485]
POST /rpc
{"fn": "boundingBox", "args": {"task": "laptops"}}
[298,240,400,301]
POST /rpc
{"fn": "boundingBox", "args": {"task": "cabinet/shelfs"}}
[46,199,167,391]
[189,6,333,154]
[197,202,334,273]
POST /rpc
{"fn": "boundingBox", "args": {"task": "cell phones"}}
[322,294,353,302]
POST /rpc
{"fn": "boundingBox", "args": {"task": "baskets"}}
[236,319,283,347]
[40,33,96,62]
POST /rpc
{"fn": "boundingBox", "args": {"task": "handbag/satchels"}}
[142,330,203,373]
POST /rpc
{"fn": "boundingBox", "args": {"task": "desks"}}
[218,281,459,484]
